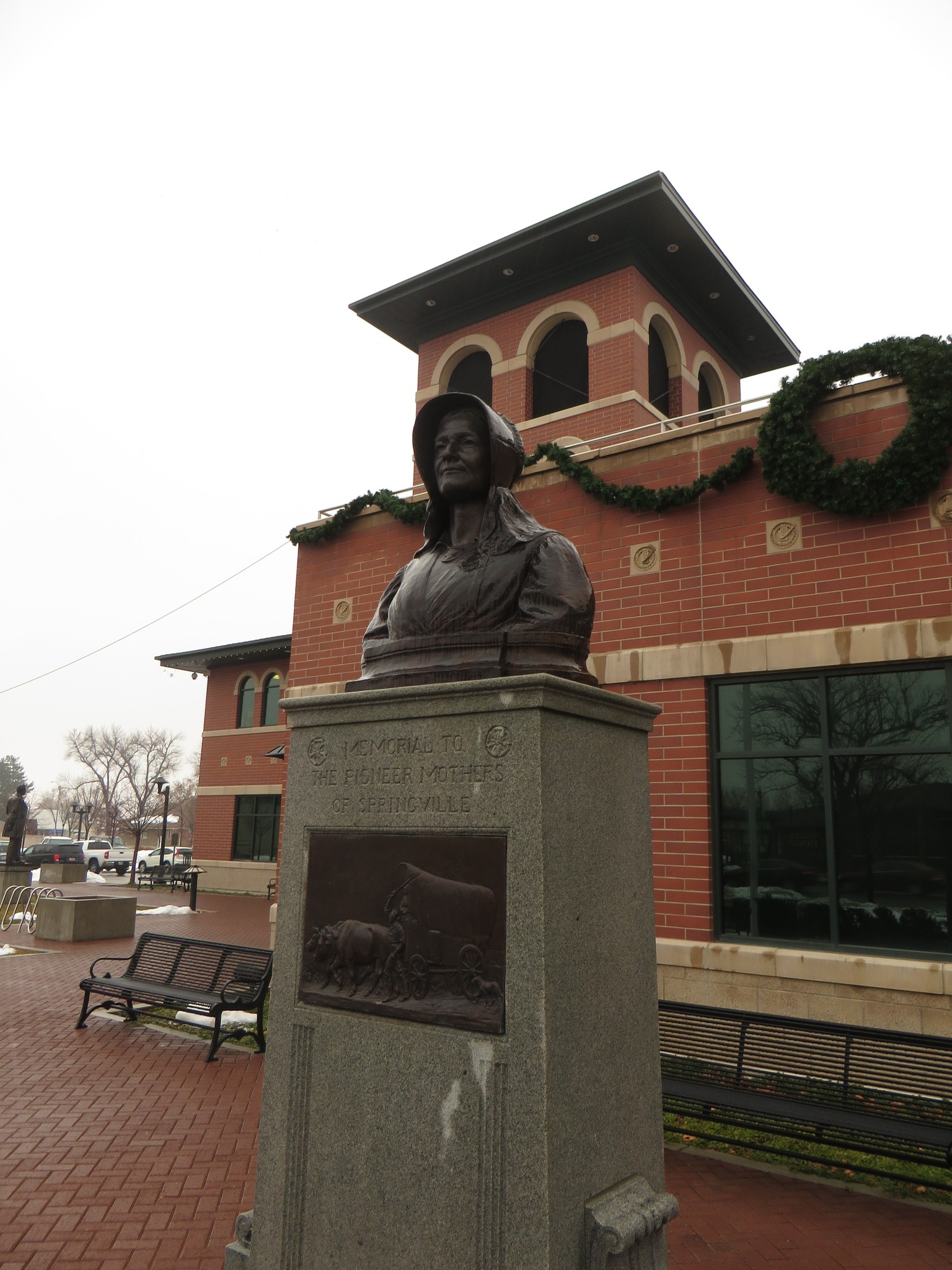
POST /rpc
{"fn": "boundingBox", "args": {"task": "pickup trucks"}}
[70,840,135,875]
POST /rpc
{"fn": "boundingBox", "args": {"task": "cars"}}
[138,846,193,875]
[0,840,10,865]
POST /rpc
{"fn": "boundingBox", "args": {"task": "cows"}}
[306,919,396,999]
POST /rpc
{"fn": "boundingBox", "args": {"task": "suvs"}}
[39,836,73,845]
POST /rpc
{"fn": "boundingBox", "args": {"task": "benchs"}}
[137,865,192,893]
[266,878,275,899]
[73,930,274,1064]
[654,998,952,1195]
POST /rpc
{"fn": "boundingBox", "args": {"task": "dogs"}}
[471,976,504,1009]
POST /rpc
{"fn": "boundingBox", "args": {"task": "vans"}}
[18,844,85,870]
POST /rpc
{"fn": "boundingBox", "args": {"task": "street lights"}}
[70,801,95,841]
[151,777,170,884]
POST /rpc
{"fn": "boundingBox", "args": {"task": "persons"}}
[1,783,28,866]
[359,392,597,670]
